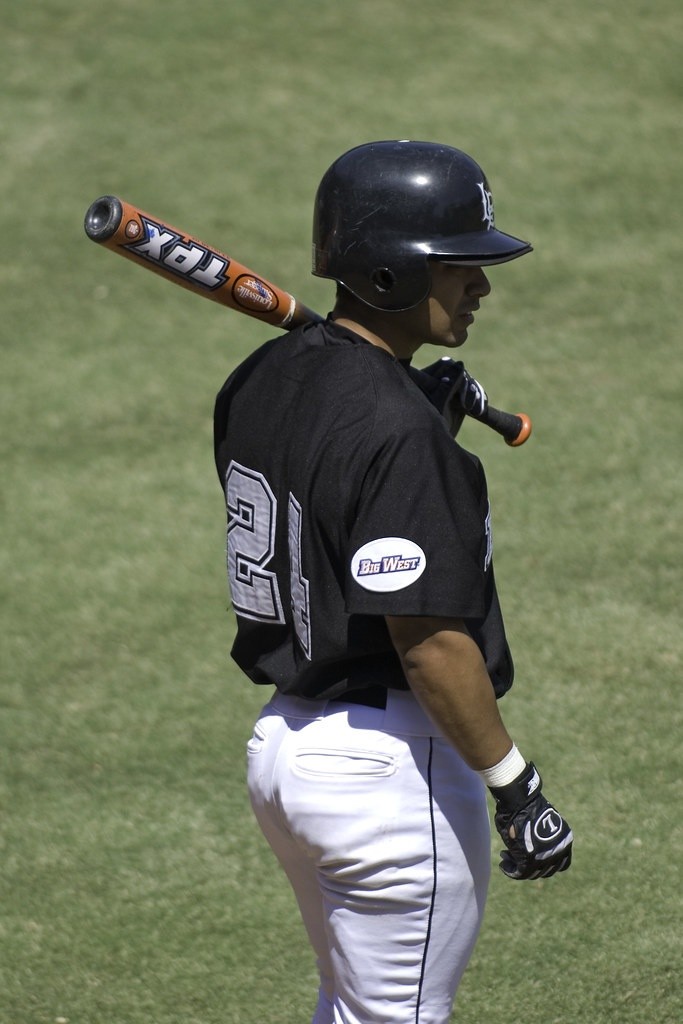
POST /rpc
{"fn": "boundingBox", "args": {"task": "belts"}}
[303,686,386,710]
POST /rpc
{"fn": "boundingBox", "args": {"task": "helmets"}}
[310,141,534,316]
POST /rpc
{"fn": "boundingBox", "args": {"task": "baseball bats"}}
[80,193,535,449]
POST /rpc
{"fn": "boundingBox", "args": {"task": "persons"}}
[214,141,572,1024]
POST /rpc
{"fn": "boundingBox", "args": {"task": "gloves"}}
[483,762,576,881]
[417,356,488,437]
